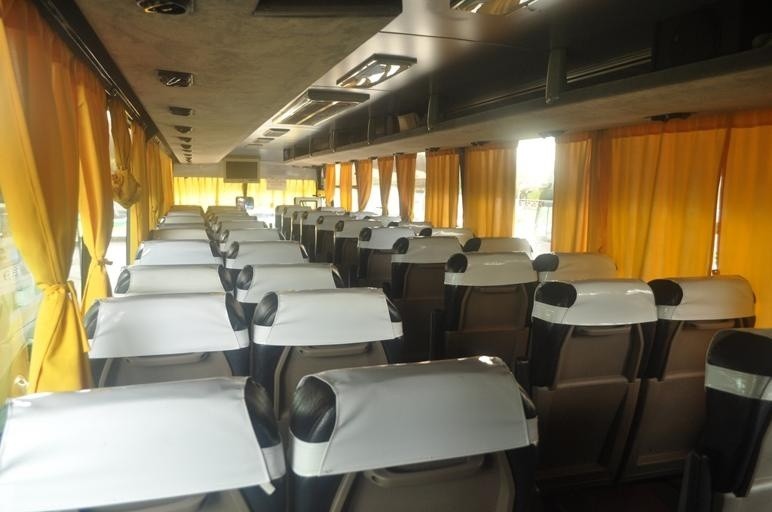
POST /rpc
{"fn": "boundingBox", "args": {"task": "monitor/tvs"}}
[223,158,261,184]
